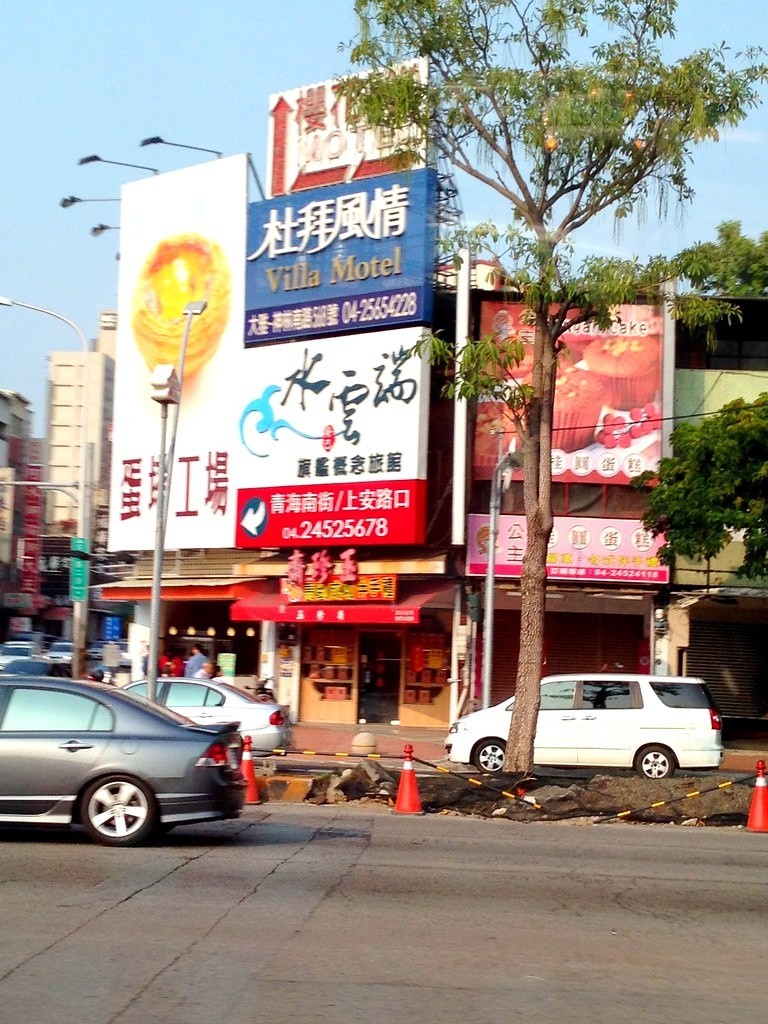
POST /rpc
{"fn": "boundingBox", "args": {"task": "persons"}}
[192,660,213,680]
[184,645,208,677]
[142,645,186,678]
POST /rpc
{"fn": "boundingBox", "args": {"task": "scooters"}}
[245,676,277,704]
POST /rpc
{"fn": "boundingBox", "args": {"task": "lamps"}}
[56,134,224,236]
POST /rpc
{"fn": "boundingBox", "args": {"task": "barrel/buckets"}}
[115,673,129,687]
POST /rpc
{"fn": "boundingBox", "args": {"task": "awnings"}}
[230,582,454,624]
[89,578,267,601]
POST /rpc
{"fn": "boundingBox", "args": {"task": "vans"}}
[443,673,725,780]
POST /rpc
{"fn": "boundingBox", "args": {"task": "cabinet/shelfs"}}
[397,628,452,729]
[299,624,358,725]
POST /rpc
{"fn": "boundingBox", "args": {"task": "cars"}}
[1,658,104,683]
[0,674,245,844]
[42,642,74,664]
[120,677,293,757]
[84,642,105,660]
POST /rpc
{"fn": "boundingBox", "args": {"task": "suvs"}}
[1,641,42,670]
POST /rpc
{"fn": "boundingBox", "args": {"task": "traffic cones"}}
[744,759,768,835]
[237,736,262,804]
[393,743,425,816]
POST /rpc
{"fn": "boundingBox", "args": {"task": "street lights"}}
[147,301,208,702]
[481,450,524,710]
[0,295,89,679]
